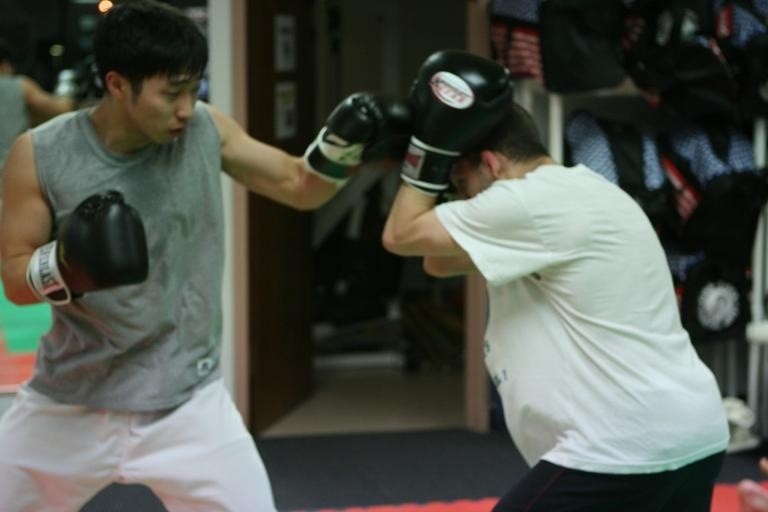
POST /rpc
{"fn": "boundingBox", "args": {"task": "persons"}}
[380,49,730,512]
[1,0,387,512]
[1,55,76,172]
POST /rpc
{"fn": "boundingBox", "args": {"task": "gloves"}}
[23,190,148,307]
[302,91,414,185]
[399,49,514,196]
